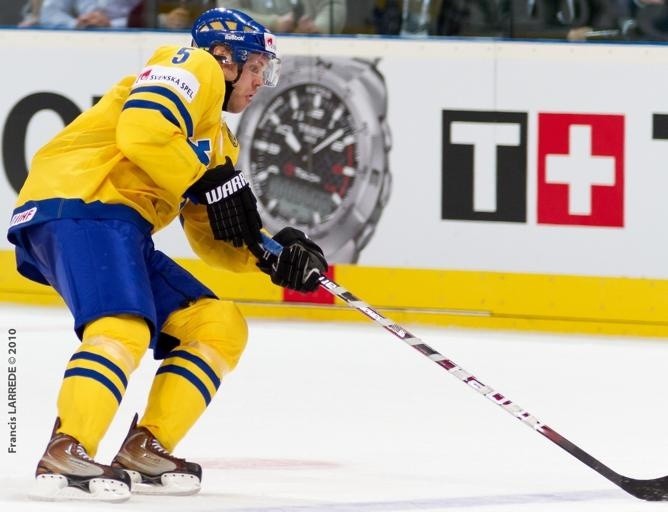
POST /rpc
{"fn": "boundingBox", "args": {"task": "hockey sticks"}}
[264,233,667,501]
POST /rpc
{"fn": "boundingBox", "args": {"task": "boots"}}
[112,410,204,487]
[33,415,133,495]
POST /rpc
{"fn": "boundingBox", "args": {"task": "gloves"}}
[196,156,264,249]
[253,222,330,292]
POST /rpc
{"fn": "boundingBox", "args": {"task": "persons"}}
[7,7,329,499]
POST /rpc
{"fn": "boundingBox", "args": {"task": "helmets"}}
[189,8,279,64]
